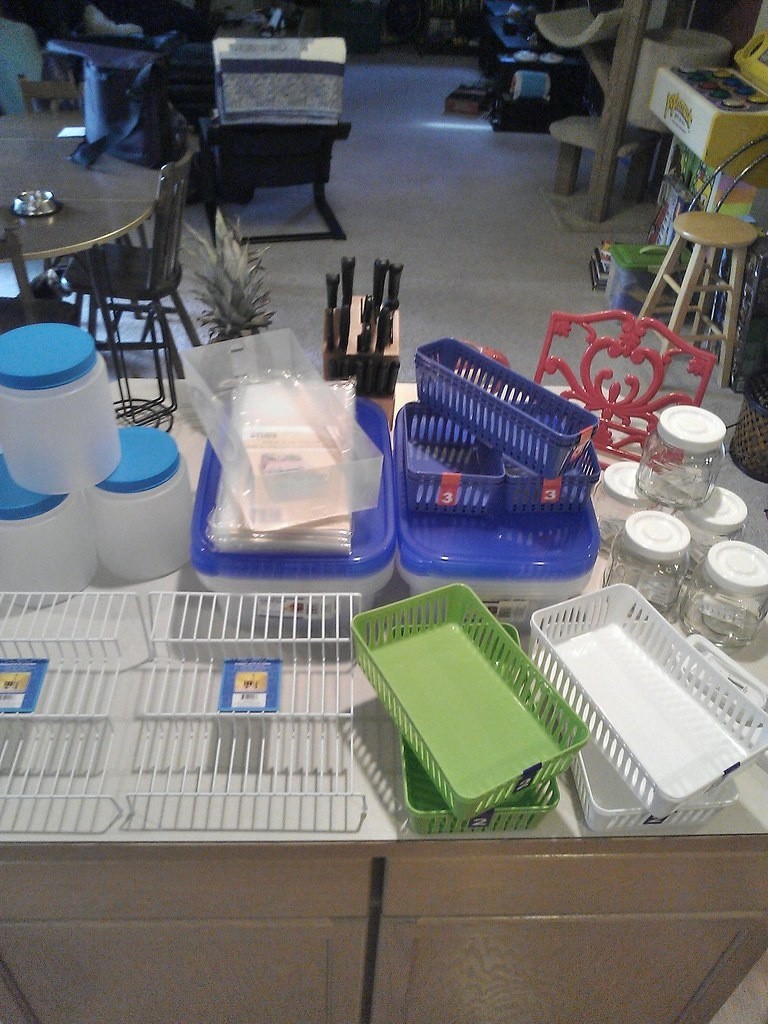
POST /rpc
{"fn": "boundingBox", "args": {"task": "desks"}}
[0,111,161,325]
[479,8,536,79]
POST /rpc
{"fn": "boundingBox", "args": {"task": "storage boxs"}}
[445,87,487,115]
[188,392,397,627]
[601,243,701,330]
[393,337,602,619]
[352,583,768,834]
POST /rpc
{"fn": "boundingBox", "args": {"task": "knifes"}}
[324,256,405,395]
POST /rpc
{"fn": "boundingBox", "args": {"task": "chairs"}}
[0,294,78,338]
[198,37,352,251]
[61,148,205,379]
[17,71,109,115]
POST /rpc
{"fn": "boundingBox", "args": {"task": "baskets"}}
[403,337,600,513]
[391,623,561,833]
[351,581,590,816]
[555,634,740,835]
[529,582,768,818]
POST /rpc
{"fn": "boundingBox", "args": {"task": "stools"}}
[479,31,503,76]
[634,212,759,388]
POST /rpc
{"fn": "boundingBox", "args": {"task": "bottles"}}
[0,322,122,495]
[591,406,768,656]
[0,453,97,609]
[89,427,193,580]
